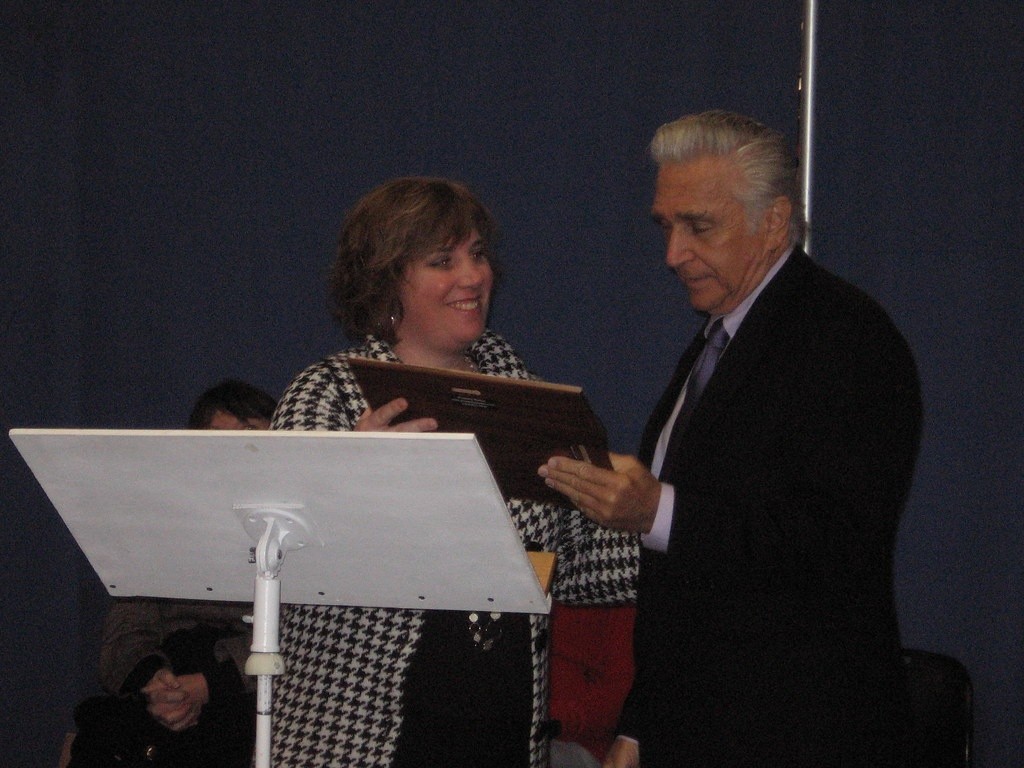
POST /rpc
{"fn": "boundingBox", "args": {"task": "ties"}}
[656,318,730,481]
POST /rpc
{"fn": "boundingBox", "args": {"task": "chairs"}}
[903,647,977,768]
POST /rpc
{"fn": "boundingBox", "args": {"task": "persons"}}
[539,109,923,768]
[61,380,279,768]
[541,600,637,768]
[255,179,640,767]
